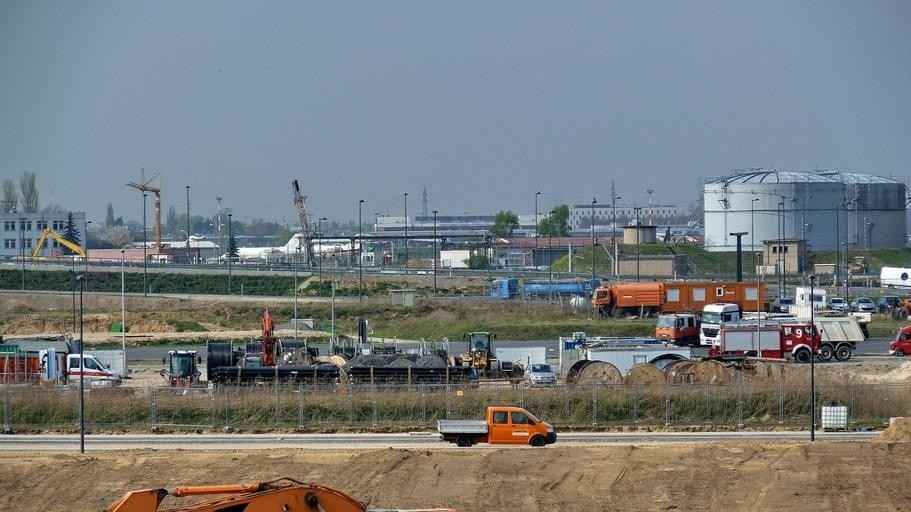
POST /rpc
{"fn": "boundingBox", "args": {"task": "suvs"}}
[876,295,904,314]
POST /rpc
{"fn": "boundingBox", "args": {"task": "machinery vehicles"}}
[159,349,206,396]
[654,285,866,364]
[848,256,870,274]
[591,283,665,318]
[884,299,911,320]
[453,331,522,386]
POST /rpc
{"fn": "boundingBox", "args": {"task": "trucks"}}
[489,277,600,301]
[888,326,910,358]
[0,349,124,389]
[436,405,557,448]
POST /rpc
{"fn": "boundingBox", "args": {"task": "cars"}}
[851,297,877,314]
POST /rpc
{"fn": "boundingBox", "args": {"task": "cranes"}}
[124,174,161,253]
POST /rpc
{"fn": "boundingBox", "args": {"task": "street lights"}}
[292,246,302,341]
[140,193,147,299]
[185,185,190,263]
[227,213,234,293]
[611,196,621,275]
[75,274,85,453]
[535,191,541,269]
[634,206,642,282]
[431,210,439,294]
[755,252,763,357]
[317,217,328,297]
[777,202,783,295]
[180,229,188,239]
[357,199,365,300]
[20,215,24,289]
[808,274,818,442]
[590,200,597,280]
[403,191,408,272]
[215,196,224,263]
[120,248,126,349]
[547,210,555,300]
[751,198,761,280]
[329,252,337,339]
[85,220,93,292]
[781,193,786,298]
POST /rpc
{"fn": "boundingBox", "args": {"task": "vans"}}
[524,363,558,388]
[879,265,911,290]
